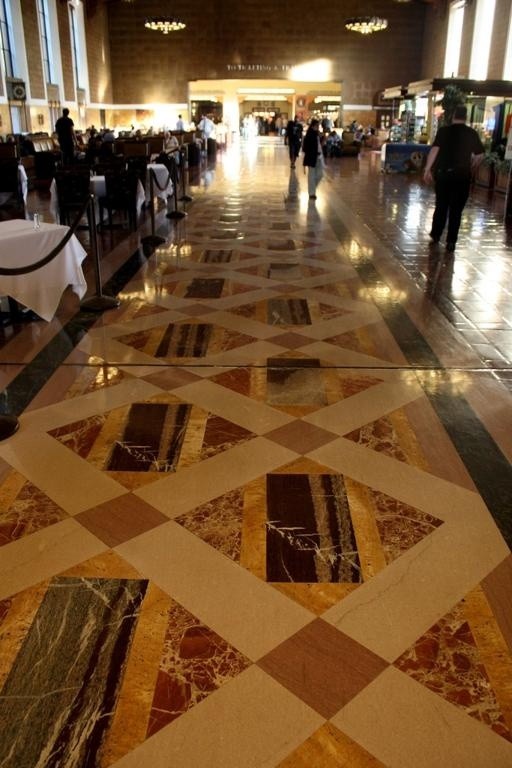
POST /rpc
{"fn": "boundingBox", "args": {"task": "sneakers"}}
[430,233,456,251]
[290,162,317,200]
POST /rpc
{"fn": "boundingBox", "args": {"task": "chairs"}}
[1,128,208,234]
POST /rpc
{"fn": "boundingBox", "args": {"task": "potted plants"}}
[472,152,510,192]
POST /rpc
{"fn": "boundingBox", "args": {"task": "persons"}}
[55,107,214,167]
[423,104,485,251]
[240,111,377,200]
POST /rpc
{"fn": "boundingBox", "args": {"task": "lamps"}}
[146,17,186,35]
[345,17,387,35]
